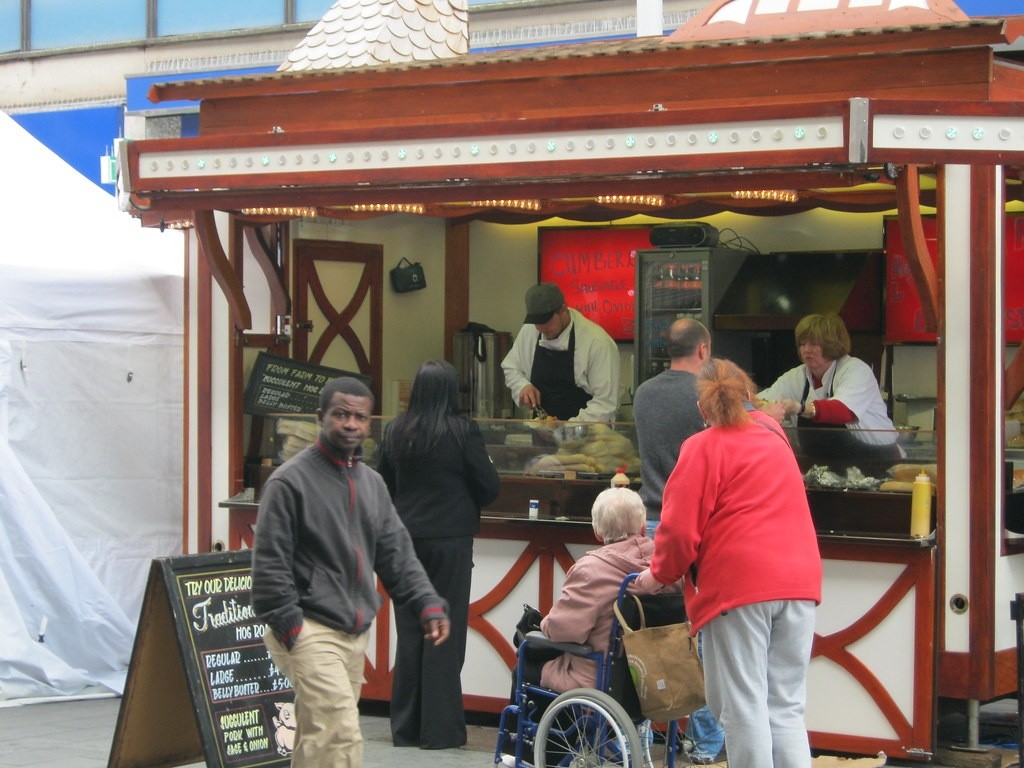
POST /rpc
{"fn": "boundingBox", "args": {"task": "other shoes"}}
[680,739,695,754]
[501,755,535,768]
[687,747,716,764]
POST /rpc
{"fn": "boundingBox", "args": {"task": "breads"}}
[880,468,938,492]
[528,416,642,473]
[1013,469,1024,488]
[1006,431,1024,448]
[276,420,378,461]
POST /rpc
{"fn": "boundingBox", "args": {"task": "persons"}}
[245,377,451,768]
[371,358,500,749]
[749,310,908,465]
[634,319,789,763]
[632,358,822,768]
[499,281,621,449]
[498,487,685,768]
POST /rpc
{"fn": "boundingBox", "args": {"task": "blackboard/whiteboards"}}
[159,549,295,768]
[244,350,375,416]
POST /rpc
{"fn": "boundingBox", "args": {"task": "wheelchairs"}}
[494,573,728,768]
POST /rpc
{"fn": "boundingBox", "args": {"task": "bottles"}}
[652,362,670,378]
[911,469,932,538]
[652,312,701,357]
[652,264,702,309]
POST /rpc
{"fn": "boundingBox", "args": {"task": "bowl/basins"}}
[894,426,922,442]
[553,425,589,447]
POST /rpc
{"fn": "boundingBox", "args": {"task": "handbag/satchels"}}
[614,594,705,724]
[513,604,563,662]
[390,258,427,293]
[461,322,496,362]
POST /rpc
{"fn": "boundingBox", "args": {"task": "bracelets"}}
[797,398,806,415]
[810,401,816,417]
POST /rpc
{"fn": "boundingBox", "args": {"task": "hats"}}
[523,283,564,324]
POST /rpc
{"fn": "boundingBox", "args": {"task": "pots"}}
[452,332,514,420]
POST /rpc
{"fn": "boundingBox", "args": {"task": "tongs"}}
[536,404,547,419]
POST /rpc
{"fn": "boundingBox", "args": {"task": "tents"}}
[0,105,229,709]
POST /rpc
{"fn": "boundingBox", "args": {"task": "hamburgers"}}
[754,399,776,408]
